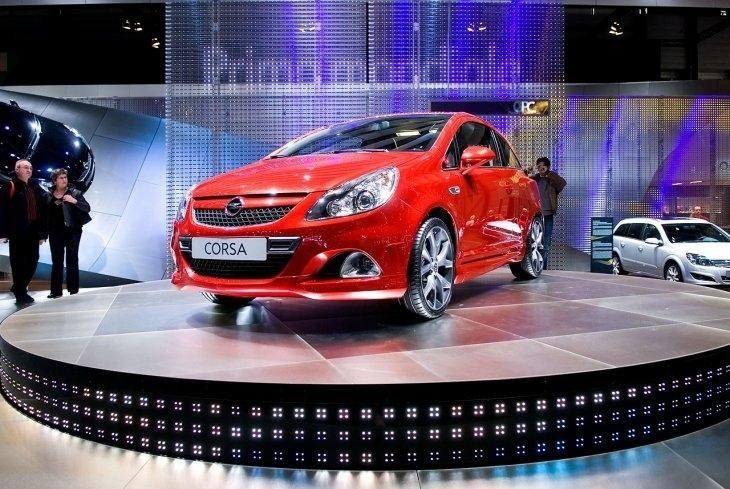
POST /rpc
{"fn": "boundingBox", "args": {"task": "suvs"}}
[611,215,730,288]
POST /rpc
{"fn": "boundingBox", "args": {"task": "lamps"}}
[465,22,488,35]
[150,36,160,50]
[609,20,623,37]
[123,16,143,32]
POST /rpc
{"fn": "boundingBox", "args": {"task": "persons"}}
[689,204,710,221]
[523,157,567,270]
[42,168,90,298]
[0,158,49,306]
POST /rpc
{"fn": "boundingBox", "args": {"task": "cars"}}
[0,99,96,199]
[167,110,546,320]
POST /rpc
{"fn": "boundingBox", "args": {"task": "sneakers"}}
[47,289,78,298]
[10,284,34,303]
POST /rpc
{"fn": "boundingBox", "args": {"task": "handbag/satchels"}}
[73,207,92,226]
